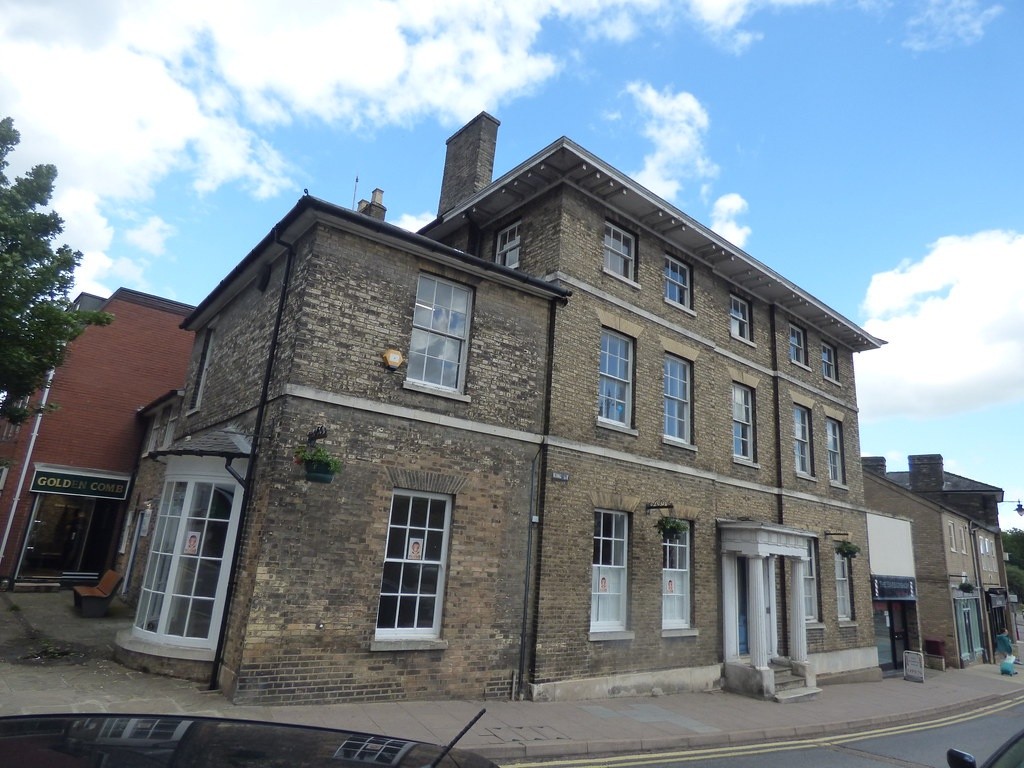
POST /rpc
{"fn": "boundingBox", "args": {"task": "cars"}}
[946,728,1024,768]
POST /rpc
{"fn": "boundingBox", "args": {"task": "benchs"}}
[73,569,124,618]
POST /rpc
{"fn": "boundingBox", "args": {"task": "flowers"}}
[294,444,344,473]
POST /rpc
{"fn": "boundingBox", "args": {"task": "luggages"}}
[1000,654,1014,676]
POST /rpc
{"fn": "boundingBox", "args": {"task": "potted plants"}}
[959,582,974,593]
[835,540,860,558]
[653,517,688,540]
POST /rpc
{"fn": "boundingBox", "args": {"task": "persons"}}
[668,580,673,591]
[411,542,420,559]
[601,577,607,588]
[187,535,197,553]
[995,628,1018,674]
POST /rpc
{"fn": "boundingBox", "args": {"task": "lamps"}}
[996,499,1024,516]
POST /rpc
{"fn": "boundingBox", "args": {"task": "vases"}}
[303,459,335,484]
